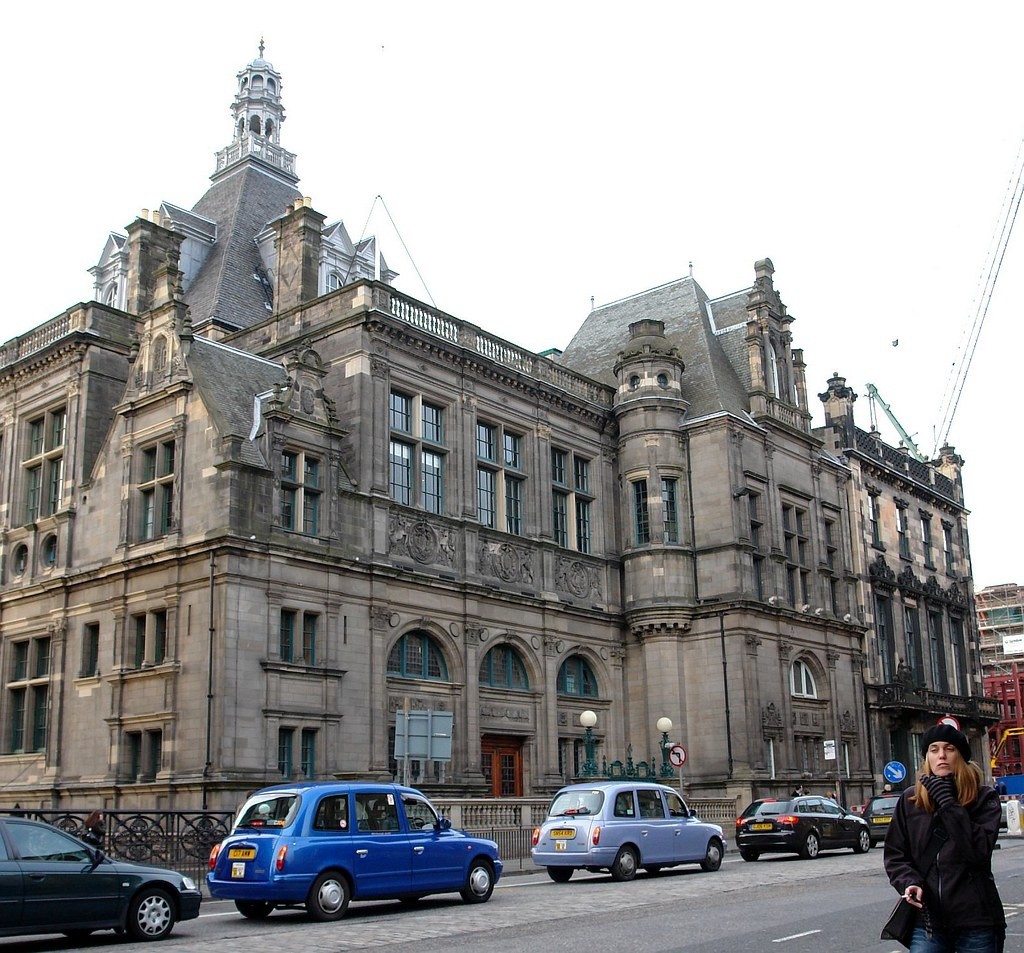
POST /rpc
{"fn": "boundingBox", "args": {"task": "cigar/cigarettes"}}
[901,894,912,898]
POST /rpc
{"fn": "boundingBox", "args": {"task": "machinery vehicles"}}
[864,381,927,465]
[990,727,1024,789]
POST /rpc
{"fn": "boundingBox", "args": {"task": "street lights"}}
[569,710,610,790]
[656,716,685,814]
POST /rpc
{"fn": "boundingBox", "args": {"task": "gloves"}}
[920,773,955,808]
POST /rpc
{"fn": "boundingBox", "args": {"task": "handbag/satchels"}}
[880,895,916,949]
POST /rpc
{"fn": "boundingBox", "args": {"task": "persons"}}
[880,724,1007,953]
[80,808,105,853]
[790,784,837,812]
[994,780,1007,803]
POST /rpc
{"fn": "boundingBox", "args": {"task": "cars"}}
[206,782,504,922]
[0,816,200,941]
[530,781,728,883]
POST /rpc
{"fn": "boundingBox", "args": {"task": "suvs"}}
[734,795,870,862]
[861,791,906,847]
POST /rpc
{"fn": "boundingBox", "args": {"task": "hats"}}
[920,725,973,764]
